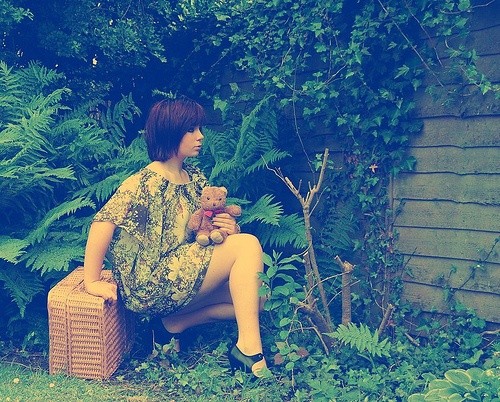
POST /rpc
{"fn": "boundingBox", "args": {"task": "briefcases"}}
[47,267,136,380]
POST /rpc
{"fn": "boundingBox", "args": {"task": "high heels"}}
[147,316,182,358]
[229,345,265,379]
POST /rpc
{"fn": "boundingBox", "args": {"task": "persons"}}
[83,98,276,385]
